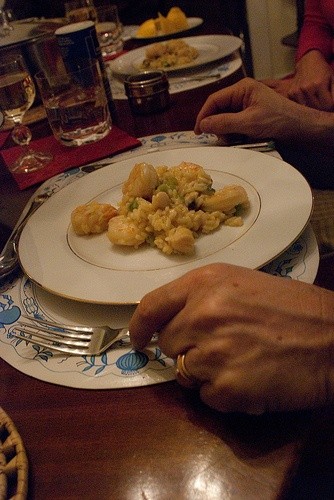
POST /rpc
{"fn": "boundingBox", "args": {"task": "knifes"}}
[81,141,275,172]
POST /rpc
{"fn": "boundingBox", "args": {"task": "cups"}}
[66,0,97,26]
[121,70,172,114]
[54,20,119,127]
[81,4,123,51]
[34,59,112,148]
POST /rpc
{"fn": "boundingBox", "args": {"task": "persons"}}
[127,0,334,414]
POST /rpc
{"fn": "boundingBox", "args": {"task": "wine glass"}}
[0,54,52,176]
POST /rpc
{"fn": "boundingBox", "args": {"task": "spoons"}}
[0,192,52,280]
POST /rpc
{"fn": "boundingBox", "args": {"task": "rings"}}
[176,353,193,384]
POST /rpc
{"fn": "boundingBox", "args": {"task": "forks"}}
[12,314,159,356]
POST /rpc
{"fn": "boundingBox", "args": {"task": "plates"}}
[130,15,204,39]
[19,146,315,304]
[110,36,242,76]
